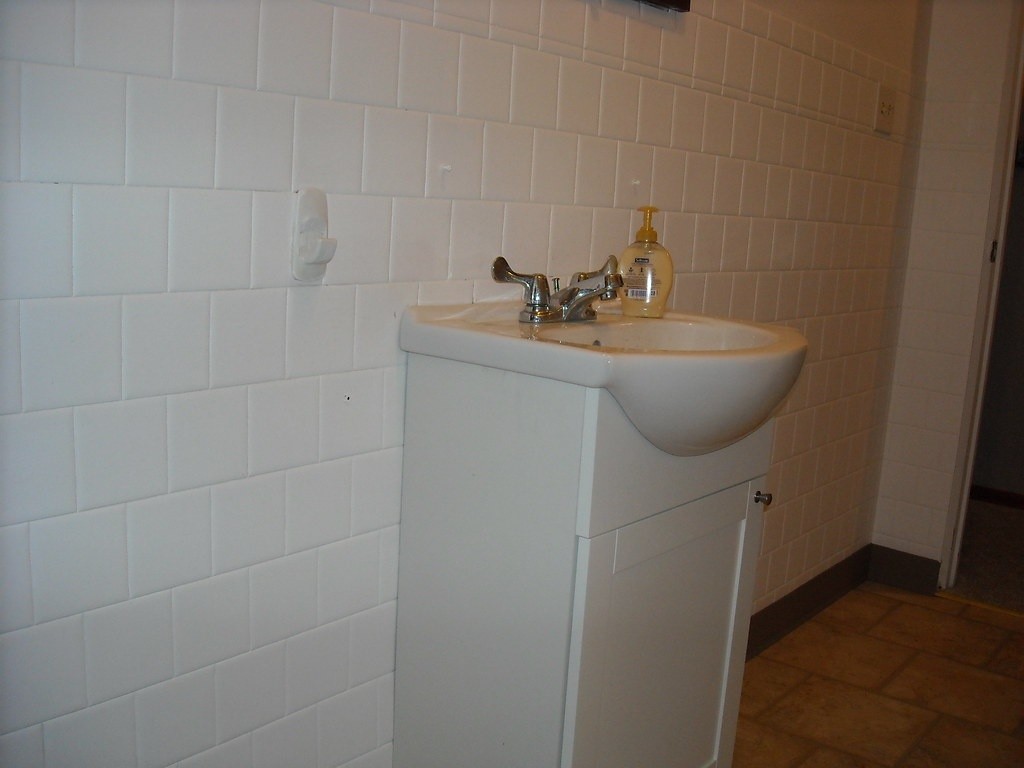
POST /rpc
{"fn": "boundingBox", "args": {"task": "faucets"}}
[549,270,625,323]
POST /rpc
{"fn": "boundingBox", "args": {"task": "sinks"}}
[397,305,806,457]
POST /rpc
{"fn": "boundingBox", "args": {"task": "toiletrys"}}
[615,207,673,317]
